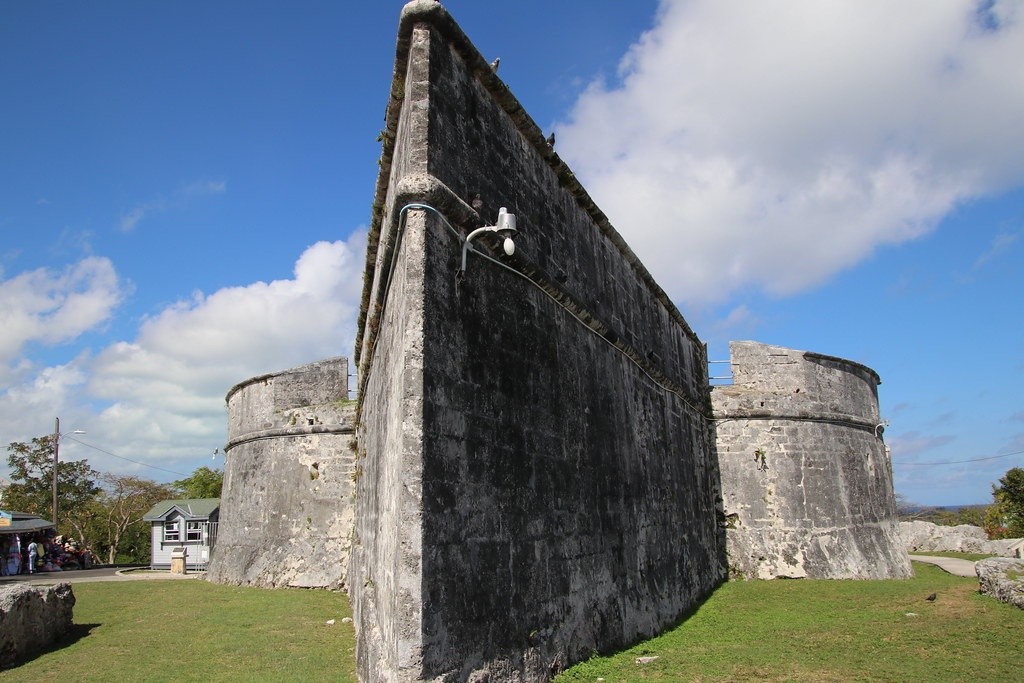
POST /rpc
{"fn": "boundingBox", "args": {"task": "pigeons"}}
[489,57,501,74]
[925,591,937,602]
[546,132,556,148]
[472,194,484,216]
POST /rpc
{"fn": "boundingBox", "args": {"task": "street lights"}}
[53,417,87,528]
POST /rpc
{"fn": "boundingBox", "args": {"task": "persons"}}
[27,538,38,574]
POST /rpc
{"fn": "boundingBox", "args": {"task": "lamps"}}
[213,448,227,463]
[874,420,891,438]
[458,208,515,276]
